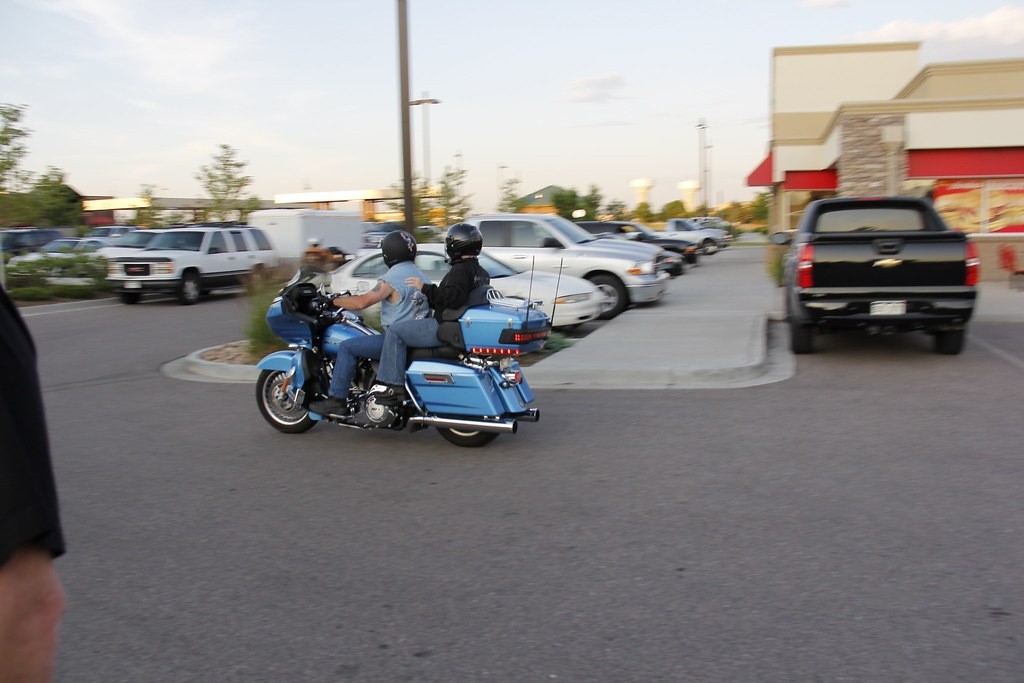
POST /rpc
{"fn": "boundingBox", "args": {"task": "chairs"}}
[995,244,1024,291]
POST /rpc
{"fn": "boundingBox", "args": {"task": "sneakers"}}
[310,395,346,415]
[374,386,406,405]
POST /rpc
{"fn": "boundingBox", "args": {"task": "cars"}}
[416,225,439,232]
[304,244,605,330]
[688,218,731,228]
[360,221,402,249]
[574,221,698,265]
[94,229,163,262]
[0,229,64,250]
[427,212,671,319]
[103,221,280,306]
[5,236,111,278]
[657,252,687,276]
[80,225,137,248]
[361,222,376,230]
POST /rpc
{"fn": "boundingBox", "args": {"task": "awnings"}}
[748,154,771,186]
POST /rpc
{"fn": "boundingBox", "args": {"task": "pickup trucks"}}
[625,218,729,255]
[769,196,980,355]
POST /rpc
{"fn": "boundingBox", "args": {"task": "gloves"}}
[314,290,351,319]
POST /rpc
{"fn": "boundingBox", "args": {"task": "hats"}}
[308,238,319,245]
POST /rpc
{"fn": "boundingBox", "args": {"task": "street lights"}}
[409,99,441,225]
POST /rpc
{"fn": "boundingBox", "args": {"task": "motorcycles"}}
[251,264,552,448]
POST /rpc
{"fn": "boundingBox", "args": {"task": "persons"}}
[358,223,490,400]
[308,230,432,415]
[0,284,66,683]
[304,239,327,267]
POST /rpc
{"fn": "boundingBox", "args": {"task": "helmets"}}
[446,223,483,260]
[381,230,417,269]
